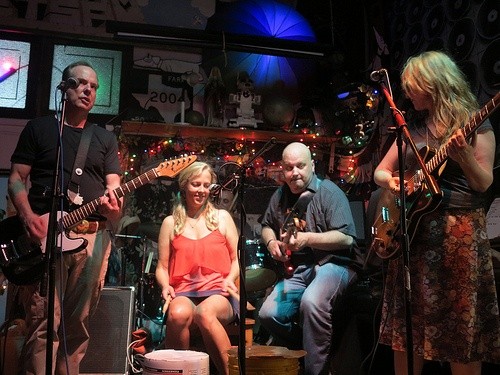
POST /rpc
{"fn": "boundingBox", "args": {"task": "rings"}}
[393,186,398,192]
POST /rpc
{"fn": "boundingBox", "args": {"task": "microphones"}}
[56,77,80,90]
[370,69,387,81]
[207,184,220,198]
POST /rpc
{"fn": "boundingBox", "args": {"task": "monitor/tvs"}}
[345,199,367,245]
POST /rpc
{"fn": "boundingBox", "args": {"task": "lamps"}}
[162,73,190,125]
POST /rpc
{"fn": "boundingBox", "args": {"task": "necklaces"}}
[425,127,442,154]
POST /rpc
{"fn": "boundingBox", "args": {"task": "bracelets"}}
[267,240,274,249]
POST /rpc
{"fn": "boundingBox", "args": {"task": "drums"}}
[143,348,209,375]
[245,238,280,293]
[228,345,307,375]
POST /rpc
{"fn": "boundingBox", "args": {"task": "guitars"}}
[0,152,198,284]
[363,92,500,260]
[267,218,307,279]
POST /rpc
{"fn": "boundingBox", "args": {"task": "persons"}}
[258,142,364,375]
[9,62,124,375]
[373,50,500,375]
[214,190,254,254]
[155,162,241,375]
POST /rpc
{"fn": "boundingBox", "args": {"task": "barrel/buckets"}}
[143,349,210,375]
[227,352,300,375]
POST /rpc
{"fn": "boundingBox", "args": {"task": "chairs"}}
[189,318,255,348]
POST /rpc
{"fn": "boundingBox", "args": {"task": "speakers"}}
[76,285,135,375]
